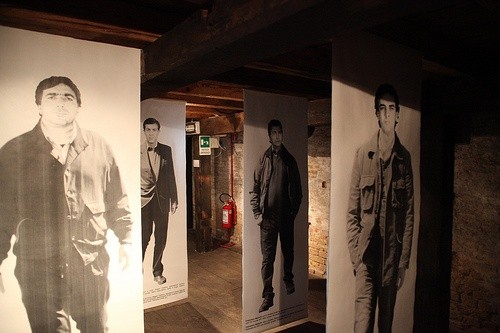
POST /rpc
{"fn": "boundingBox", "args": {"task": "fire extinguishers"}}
[221,201,233,229]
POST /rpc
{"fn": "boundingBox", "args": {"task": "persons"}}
[140,117,179,285]
[345,84,415,333]
[0,75,135,333]
[249,119,303,313]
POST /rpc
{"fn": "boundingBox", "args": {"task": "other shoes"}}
[257,297,273,313]
[286,281,295,295]
[153,275,168,284]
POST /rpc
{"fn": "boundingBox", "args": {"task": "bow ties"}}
[147,146,159,152]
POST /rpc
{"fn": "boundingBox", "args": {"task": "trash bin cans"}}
[196,218,213,253]
